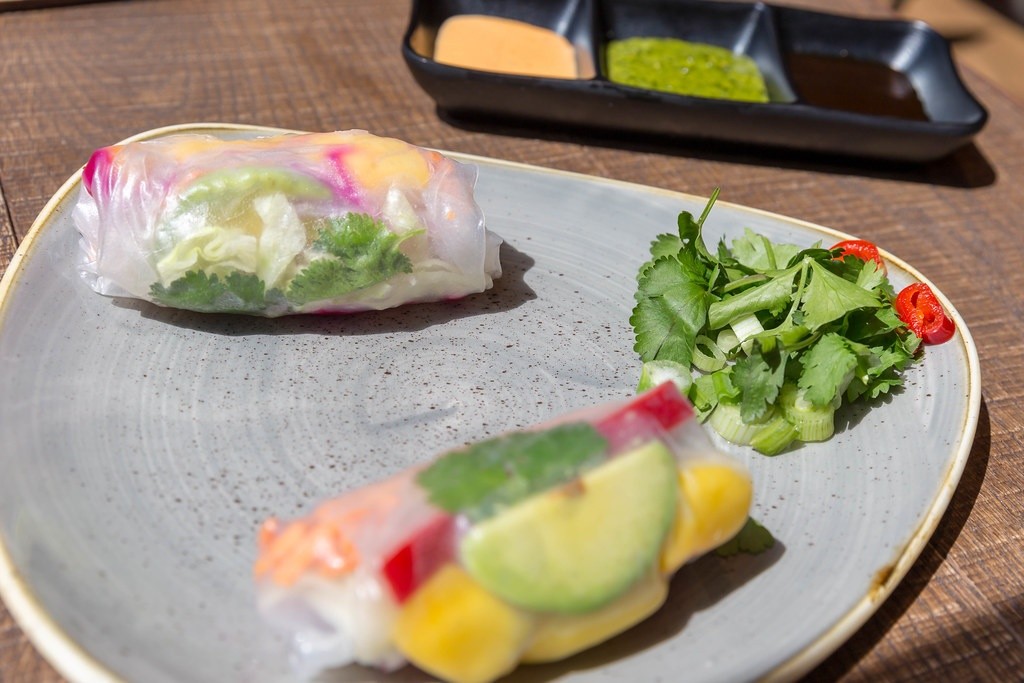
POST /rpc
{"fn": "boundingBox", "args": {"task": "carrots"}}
[255,477,412,585]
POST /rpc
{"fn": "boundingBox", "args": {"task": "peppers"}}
[825,239,885,272]
[380,511,457,606]
[595,380,696,447]
[896,283,955,345]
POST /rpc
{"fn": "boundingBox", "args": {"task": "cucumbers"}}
[390,460,750,683]
[460,438,680,608]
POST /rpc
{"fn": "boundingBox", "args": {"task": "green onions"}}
[636,311,835,455]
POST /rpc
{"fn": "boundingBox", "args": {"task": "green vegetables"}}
[149,209,429,321]
[628,186,922,423]
[411,419,607,515]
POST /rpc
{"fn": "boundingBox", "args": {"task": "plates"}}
[0,121,982,683]
[400,0,989,162]
